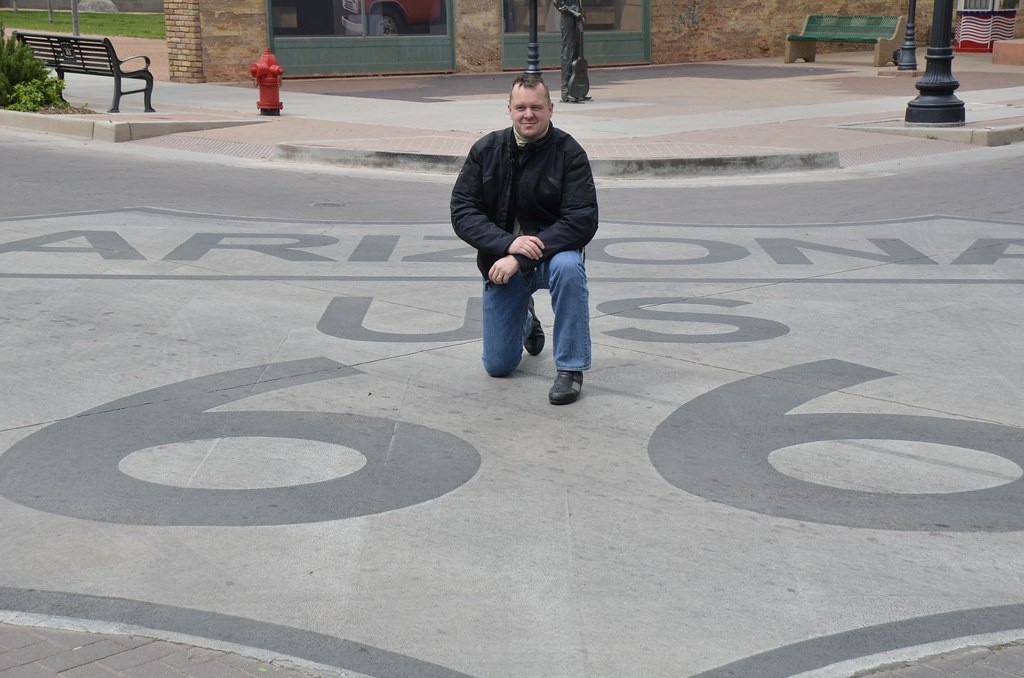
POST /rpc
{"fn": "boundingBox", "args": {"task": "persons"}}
[449,76,598,404]
[553,0,593,101]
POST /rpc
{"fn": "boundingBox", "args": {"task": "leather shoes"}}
[524,297,545,355]
[548,370,583,404]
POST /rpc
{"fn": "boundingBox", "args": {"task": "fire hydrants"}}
[250,47,286,117]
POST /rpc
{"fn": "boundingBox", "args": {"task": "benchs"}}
[784,14,907,67]
[13,30,156,113]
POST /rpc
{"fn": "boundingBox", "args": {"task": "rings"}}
[498,276,503,278]
